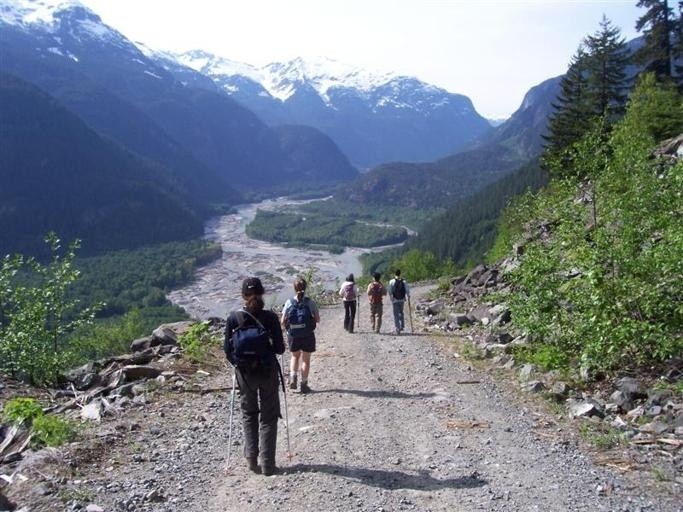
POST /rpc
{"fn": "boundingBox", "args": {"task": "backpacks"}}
[283,296,316,337]
[229,310,273,371]
[343,283,355,300]
[392,278,406,301]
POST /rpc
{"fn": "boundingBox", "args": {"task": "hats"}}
[241,277,265,296]
[371,271,381,278]
[392,269,401,275]
[345,273,354,282]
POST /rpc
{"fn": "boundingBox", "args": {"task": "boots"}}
[299,380,311,393]
[286,375,297,389]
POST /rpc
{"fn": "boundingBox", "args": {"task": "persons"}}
[364,271,386,335]
[223,277,286,477]
[337,273,359,333]
[387,268,409,335]
[280,275,319,394]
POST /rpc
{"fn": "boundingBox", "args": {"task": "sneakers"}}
[245,456,258,472]
